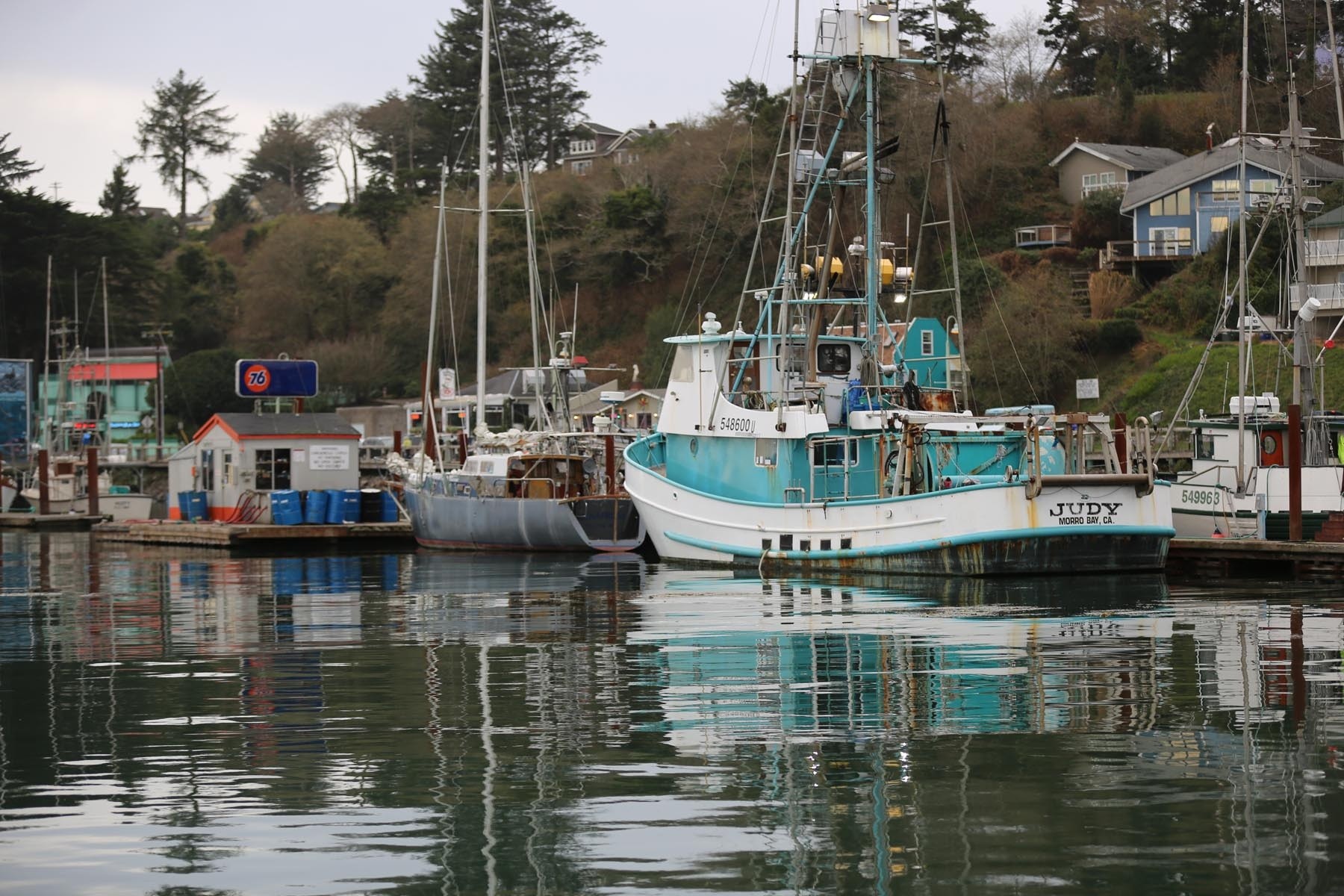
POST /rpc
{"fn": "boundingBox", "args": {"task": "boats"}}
[1157,472,1270,557]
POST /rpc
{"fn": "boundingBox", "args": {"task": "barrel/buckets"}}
[178,486,208,520]
[271,542,404,596]
[177,557,210,600]
[268,487,401,527]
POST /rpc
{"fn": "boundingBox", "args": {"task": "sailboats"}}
[413,556,1179,895]
[1187,0,1344,540]
[0,250,149,523]
[398,2,649,549]
[622,0,1174,566]
